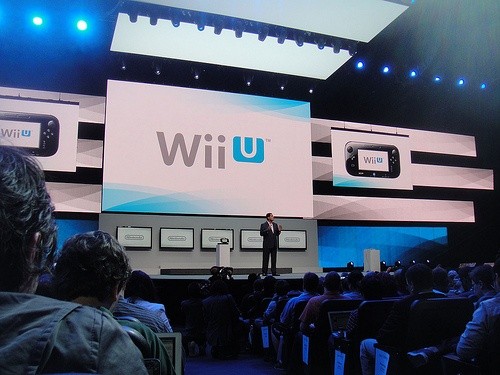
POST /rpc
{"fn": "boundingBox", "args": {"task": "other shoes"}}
[262,272,267,275]
[272,273,280,275]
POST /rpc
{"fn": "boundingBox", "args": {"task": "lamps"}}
[274,29,288,48]
[312,37,328,53]
[292,32,308,48]
[147,6,162,27]
[193,11,209,32]
[343,258,433,273]
[211,18,226,37]
[166,8,184,30]
[329,40,344,55]
[256,25,273,43]
[348,42,363,58]
[232,23,247,41]
[125,2,141,26]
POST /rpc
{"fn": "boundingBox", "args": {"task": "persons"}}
[112,270,173,332]
[0,144,150,375]
[260,213,283,276]
[207,266,233,296]
[180,282,208,357]
[239,262,500,375]
[51,230,176,375]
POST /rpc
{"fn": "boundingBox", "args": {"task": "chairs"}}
[114,314,161,375]
[263,295,500,375]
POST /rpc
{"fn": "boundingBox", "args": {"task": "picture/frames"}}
[155,332,183,375]
